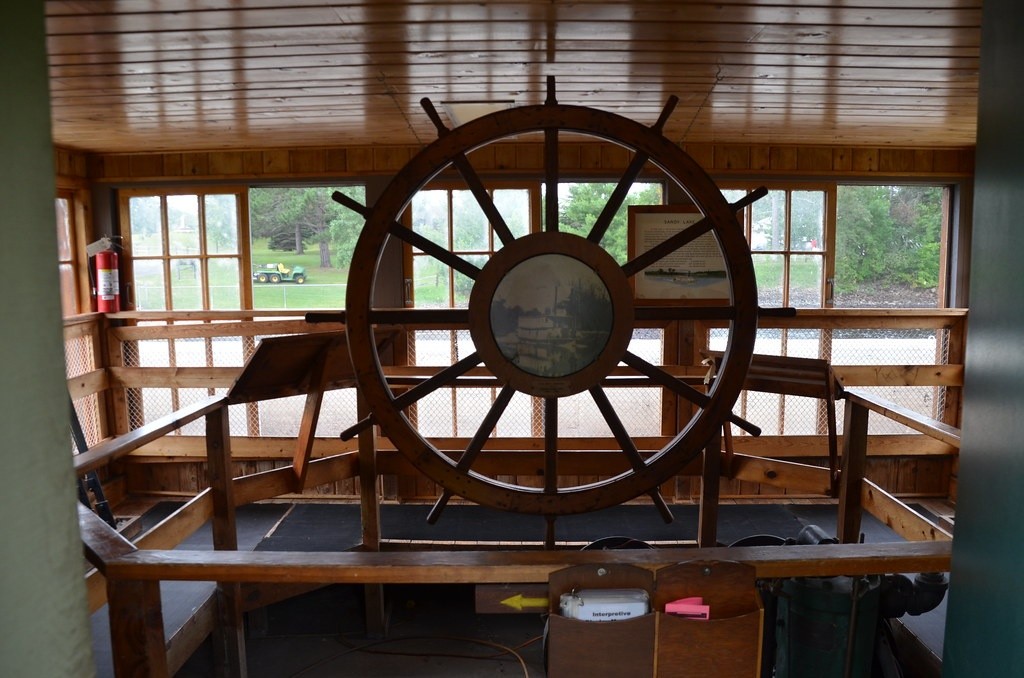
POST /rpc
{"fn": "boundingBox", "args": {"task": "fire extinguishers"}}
[87,234,124,312]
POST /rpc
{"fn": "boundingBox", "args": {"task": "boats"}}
[513,288,581,346]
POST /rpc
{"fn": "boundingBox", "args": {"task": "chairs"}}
[277,263,290,279]
